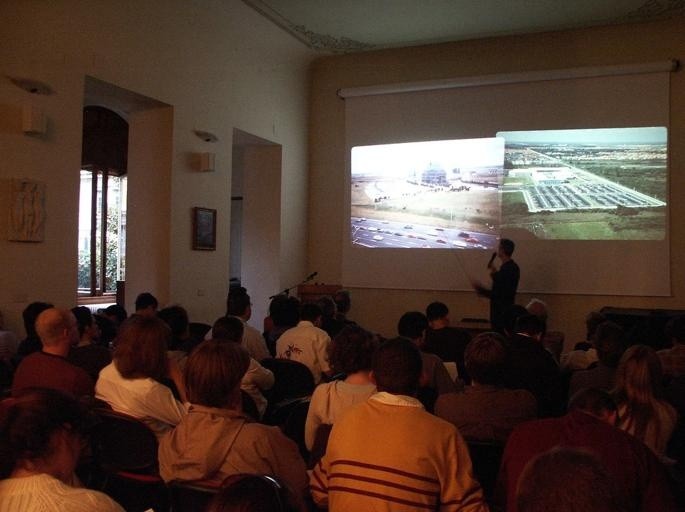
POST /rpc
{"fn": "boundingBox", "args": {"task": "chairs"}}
[239,388,259,423]
[110,472,167,512]
[28,387,79,430]
[161,379,183,403]
[90,409,157,471]
[269,401,311,463]
[463,436,504,497]
[515,447,620,512]
[169,479,221,512]
[209,471,296,512]
[258,357,316,403]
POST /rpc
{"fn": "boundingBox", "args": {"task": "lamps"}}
[191,151,216,173]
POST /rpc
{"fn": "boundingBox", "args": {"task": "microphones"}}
[305,271,318,281]
[487,252,497,269]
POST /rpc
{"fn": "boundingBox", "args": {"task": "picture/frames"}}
[193,206,217,250]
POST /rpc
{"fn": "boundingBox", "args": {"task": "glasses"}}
[249,303,254,306]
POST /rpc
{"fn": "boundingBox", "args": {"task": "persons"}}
[305,330,391,460]
[655,319,684,375]
[311,339,490,512]
[503,307,532,339]
[499,317,565,410]
[263,296,299,356]
[68,307,109,374]
[435,334,537,444]
[316,297,341,338]
[423,302,464,359]
[276,304,338,389]
[334,291,386,351]
[398,313,460,397]
[95,316,194,472]
[526,301,564,353]
[158,308,200,356]
[1,391,125,512]
[473,238,520,333]
[202,289,273,365]
[572,325,627,371]
[158,342,311,512]
[575,313,612,352]
[207,473,289,512]
[135,293,158,318]
[16,304,55,357]
[493,389,675,512]
[572,347,679,472]
[211,317,275,422]
[11,309,100,402]
[96,308,127,364]
